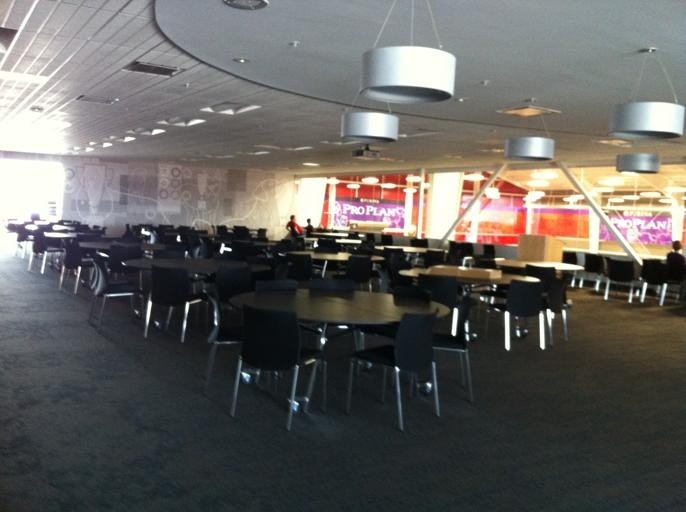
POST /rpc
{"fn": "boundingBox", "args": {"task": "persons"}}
[666,241,686,282]
[285,215,303,240]
[304,219,313,238]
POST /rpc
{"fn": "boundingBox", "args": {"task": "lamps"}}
[607,47,685,140]
[359,1,456,104]
[615,142,660,174]
[340,94,399,143]
[503,99,555,162]
